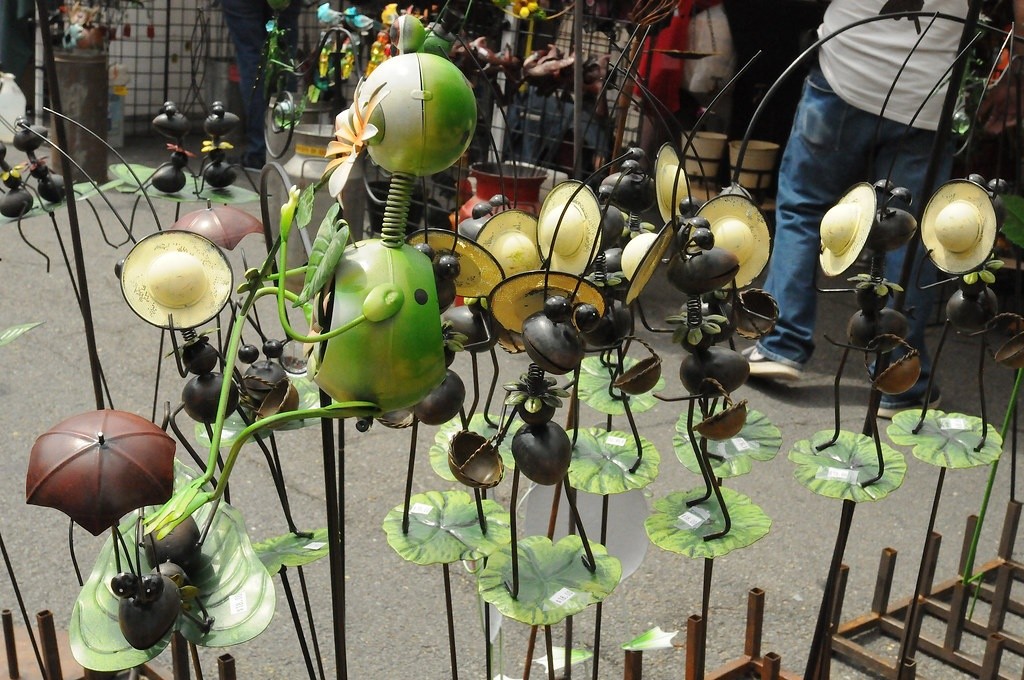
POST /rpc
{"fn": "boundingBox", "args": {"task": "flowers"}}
[248,1,610,175]
[52,0,153,52]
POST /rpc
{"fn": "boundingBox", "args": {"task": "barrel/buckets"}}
[279,124,366,300]
[453,165,550,306]
[728,140,779,189]
[679,131,725,178]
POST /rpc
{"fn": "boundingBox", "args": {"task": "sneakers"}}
[877,381,942,418]
[741,346,802,380]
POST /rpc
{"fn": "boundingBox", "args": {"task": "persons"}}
[742,0,1023,416]
[221,0,302,172]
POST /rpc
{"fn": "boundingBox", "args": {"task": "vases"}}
[280,124,365,296]
[51,48,108,183]
[453,165,548,308]
[260,95,331,246]
[683,131,727,183]
[731,142,779,189]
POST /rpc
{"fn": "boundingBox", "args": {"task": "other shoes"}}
[228,152,267,171]
